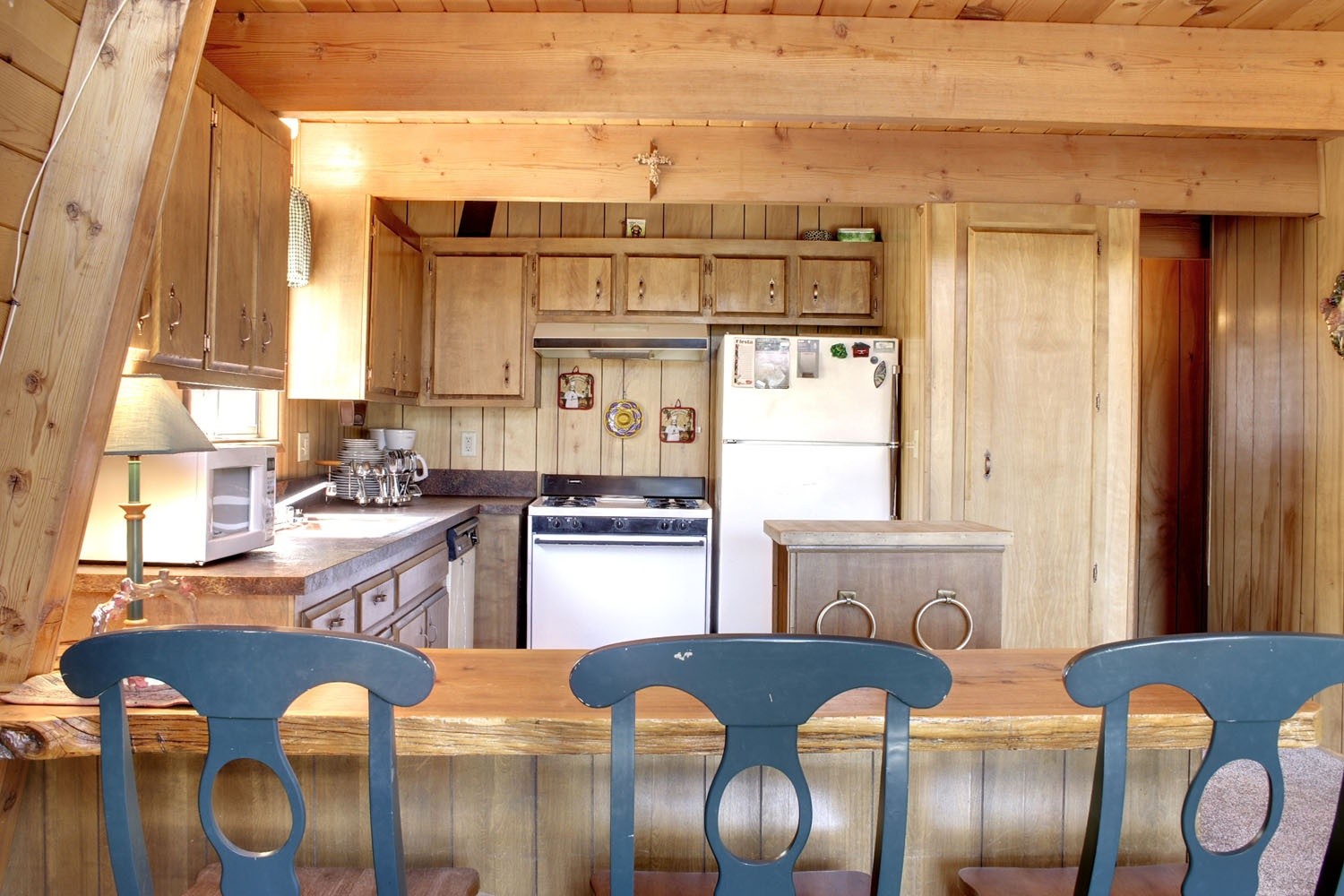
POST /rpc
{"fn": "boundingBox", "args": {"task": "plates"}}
[331,438,388,500]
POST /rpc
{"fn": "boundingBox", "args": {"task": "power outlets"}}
[461,432,477,456]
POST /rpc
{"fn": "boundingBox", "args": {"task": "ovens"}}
[525,534,712,650]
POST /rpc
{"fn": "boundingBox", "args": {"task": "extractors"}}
[530,320,711,362]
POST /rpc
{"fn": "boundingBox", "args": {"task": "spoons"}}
[351,451,417,507]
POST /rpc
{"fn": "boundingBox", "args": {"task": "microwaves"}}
[77,446,277,567]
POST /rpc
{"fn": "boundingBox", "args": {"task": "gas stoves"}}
[527,473,713,536]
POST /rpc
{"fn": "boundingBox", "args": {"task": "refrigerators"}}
[713,333,900,634]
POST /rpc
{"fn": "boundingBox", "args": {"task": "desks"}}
[0,649,1320,896]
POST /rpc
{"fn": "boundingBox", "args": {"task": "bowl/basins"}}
[383,429,417,450]
[368,428,387,450]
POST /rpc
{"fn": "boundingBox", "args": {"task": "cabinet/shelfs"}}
[533,239,701,319]
[205,94,294,394]
[298,196,420,407]
[927,201,1102,650]
[297,536,450,650]
[127,83,212,393]
[422,235,535,407]
[706,238,883,327]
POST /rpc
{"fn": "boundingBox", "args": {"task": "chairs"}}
[61,623,481,896]
[957,631,1344,896]
[568,634,952,896]
